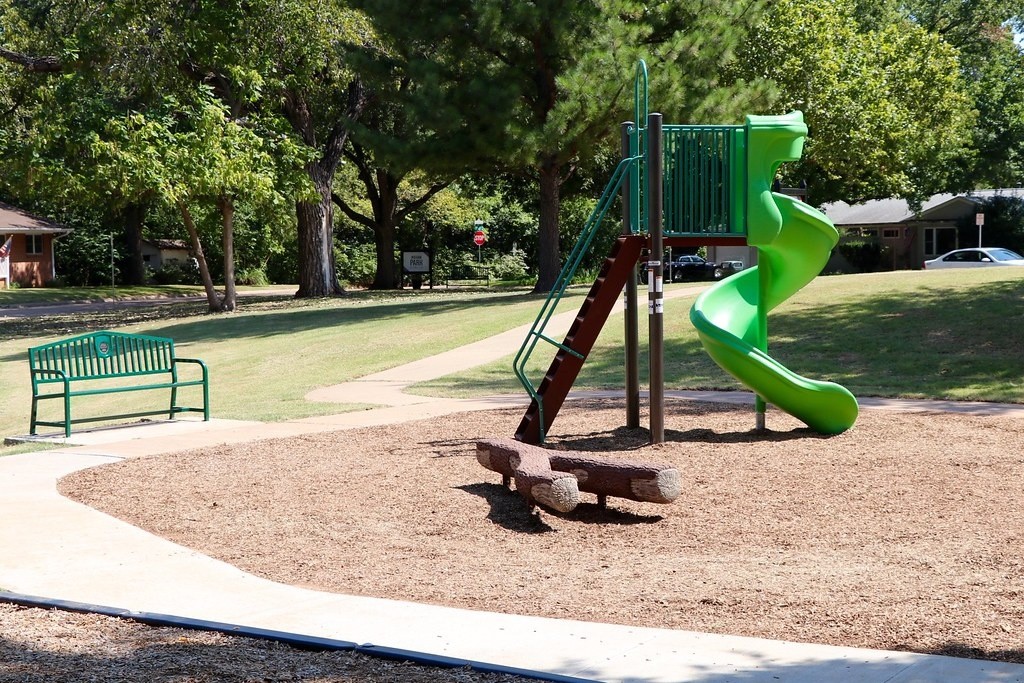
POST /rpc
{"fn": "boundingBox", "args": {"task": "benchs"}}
[27,330,207,437]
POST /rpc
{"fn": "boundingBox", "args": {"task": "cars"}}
[921,248,1024,270]
[639,254,725,283]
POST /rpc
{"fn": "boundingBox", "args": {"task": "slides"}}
[692,107,860,434]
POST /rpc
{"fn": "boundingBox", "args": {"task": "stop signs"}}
[473,231,486,245]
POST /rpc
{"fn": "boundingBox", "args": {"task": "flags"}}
[0,236,12,259]
